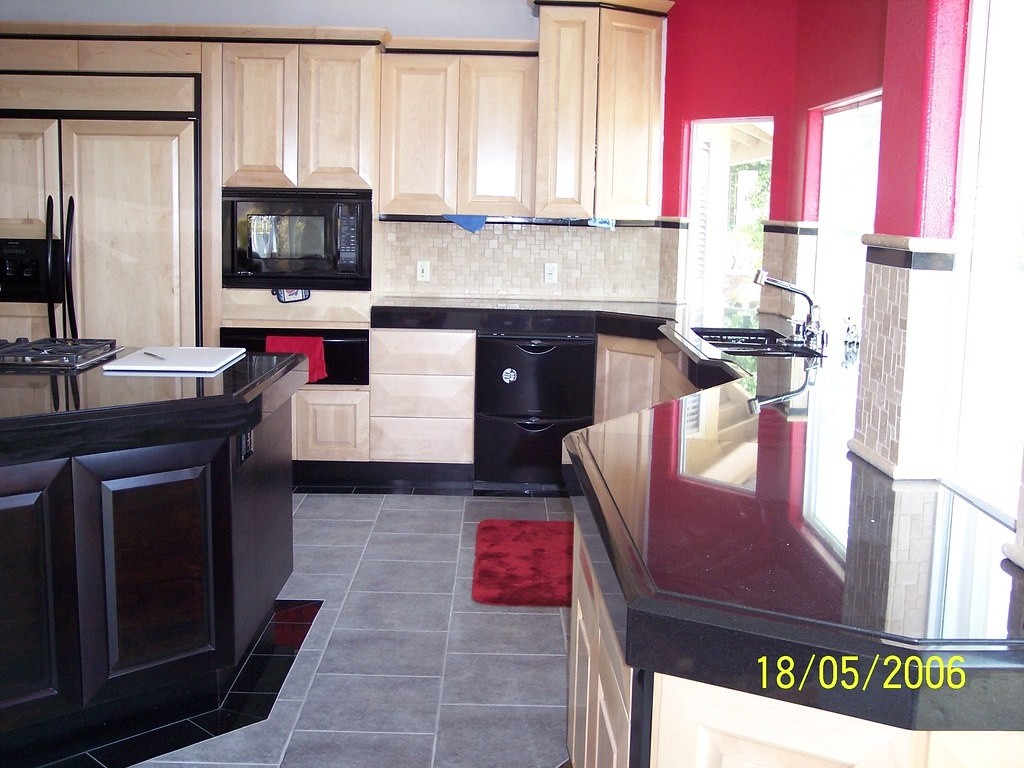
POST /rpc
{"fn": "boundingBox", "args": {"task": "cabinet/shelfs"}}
[1,0,676,490]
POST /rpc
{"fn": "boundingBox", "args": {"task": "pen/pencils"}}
[144,352,165,361]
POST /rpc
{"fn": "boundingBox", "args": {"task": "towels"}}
[265,336,328,383]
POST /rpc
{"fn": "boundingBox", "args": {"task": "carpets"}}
[472,520,575,606]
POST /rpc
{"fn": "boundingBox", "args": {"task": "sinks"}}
[708,342,826,358]
[690,327,790,341]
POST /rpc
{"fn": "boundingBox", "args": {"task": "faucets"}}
[754,268,827,346]
[743,357,824,427]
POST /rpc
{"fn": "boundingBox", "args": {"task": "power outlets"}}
[545,263,557,283]
[417,261,429,281]
